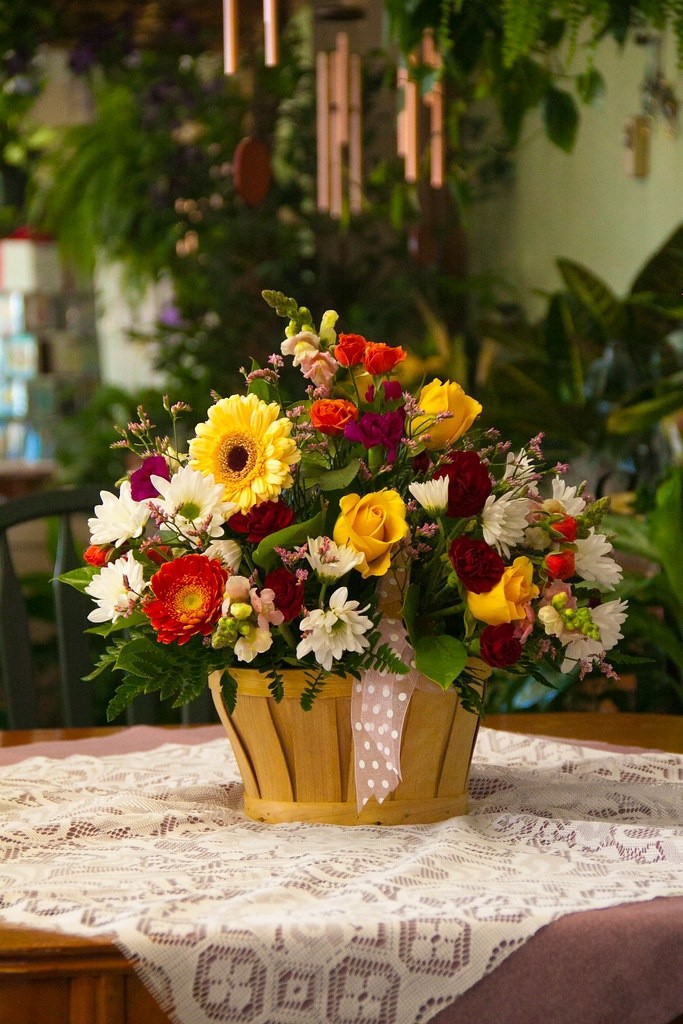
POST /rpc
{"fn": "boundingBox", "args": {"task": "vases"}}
[205,667,479,824]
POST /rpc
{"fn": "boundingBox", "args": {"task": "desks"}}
[0,718,682,1024]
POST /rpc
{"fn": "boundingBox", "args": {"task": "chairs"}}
[0,491,103,732]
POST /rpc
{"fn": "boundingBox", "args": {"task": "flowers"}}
[49,286,631,716]
